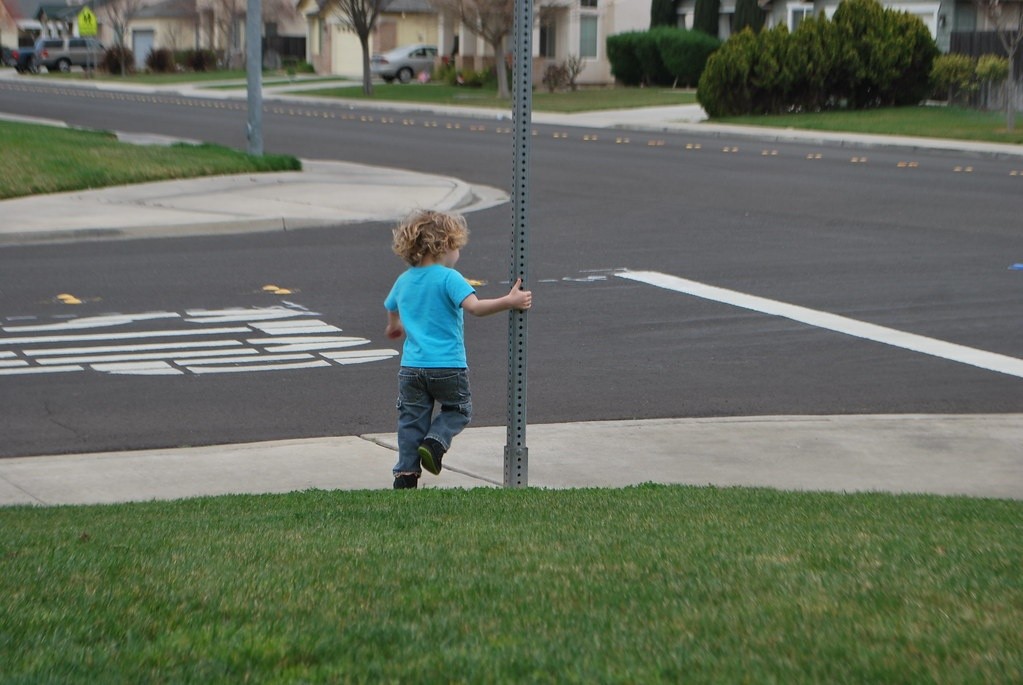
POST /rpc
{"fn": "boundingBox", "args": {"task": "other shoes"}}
[419,438,442,475]
[394,475,418,489]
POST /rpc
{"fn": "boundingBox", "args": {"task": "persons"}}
[384,210,532,489]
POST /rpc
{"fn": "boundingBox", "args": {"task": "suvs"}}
[34,36,109,73]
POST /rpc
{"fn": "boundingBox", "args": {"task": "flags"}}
[418,69,430,83]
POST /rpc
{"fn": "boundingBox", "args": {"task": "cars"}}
[369,43,455,85]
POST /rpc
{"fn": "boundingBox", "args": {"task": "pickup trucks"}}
[3,45,39,74]
